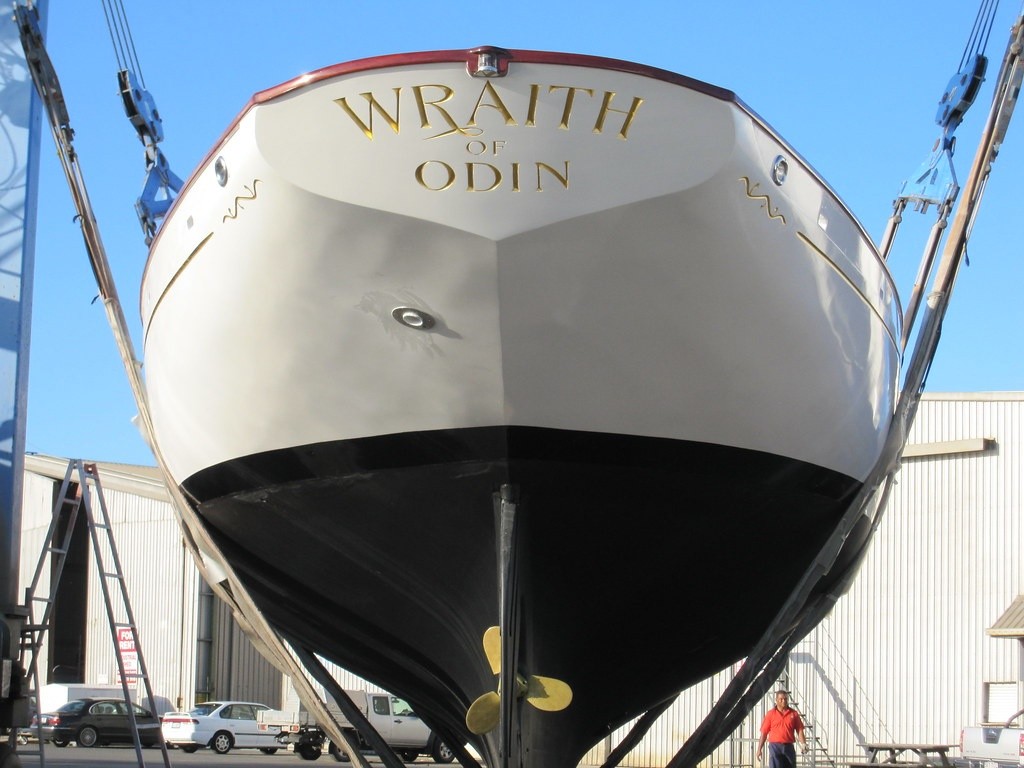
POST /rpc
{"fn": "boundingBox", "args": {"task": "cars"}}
[162,700,290,755]
[29,698,160,748]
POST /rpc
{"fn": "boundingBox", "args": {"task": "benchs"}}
[849,762,956,768]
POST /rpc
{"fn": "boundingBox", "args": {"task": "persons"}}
[757,690,809,768]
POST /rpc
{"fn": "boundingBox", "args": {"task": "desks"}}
[868,743,950,768]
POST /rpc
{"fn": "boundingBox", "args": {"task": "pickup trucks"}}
[256,688,455,765]
[959,727,1024,768]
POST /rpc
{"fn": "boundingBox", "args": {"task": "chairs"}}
[98,705,104,713]
[104,706,114,714]
